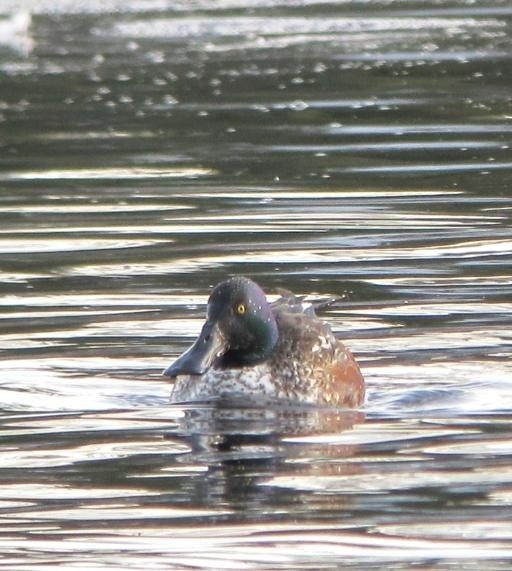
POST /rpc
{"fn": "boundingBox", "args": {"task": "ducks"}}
[162,276,365,405]
